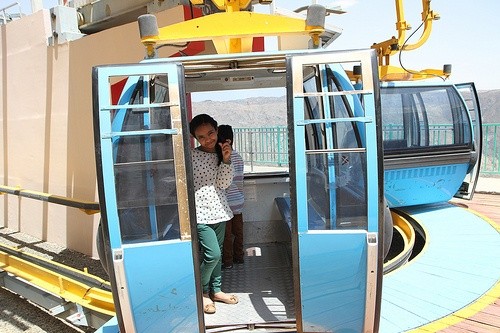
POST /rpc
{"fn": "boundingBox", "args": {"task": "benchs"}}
[274,193,329,232]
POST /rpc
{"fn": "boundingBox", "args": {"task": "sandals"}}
[200,293,216,314]
[210,292,238,305]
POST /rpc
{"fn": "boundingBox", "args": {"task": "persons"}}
[215,124,246,271]
[187,113,239,314]
